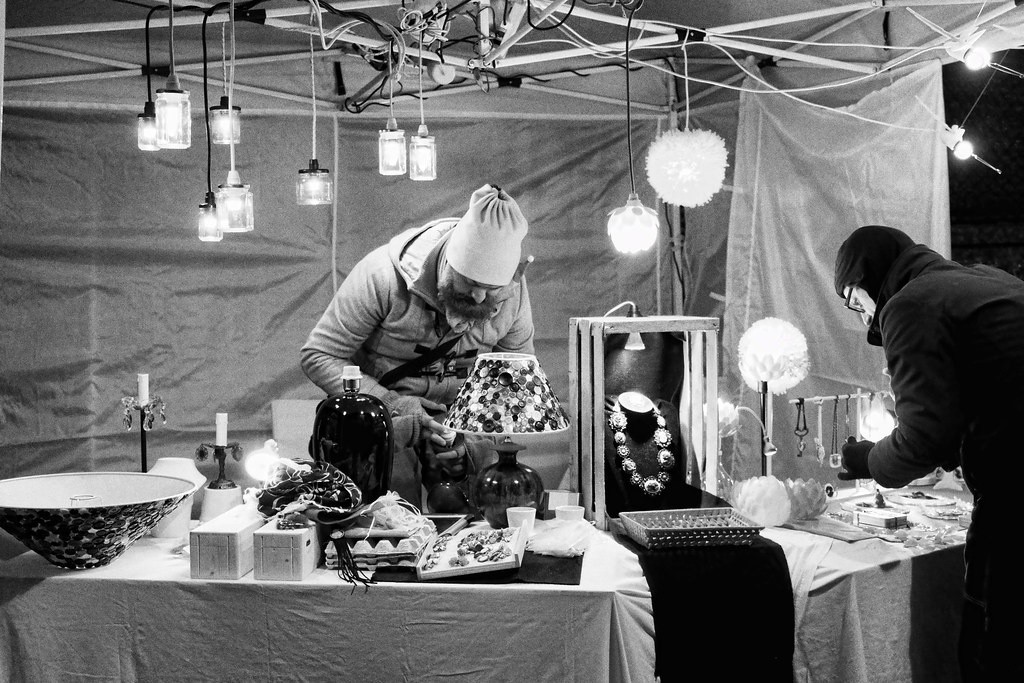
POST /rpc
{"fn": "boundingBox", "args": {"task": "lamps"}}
[941,131,973,160]
[944,41,988,71]
[702,318,898,478]
[138,0,437,242]
[607,3,730,255]
[603,301,644,348]
[245,439,311,482]
[442,351,571,529]
[0,472,197,570]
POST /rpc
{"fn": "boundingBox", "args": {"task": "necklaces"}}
[611,399,676,496]
[844,393,850,442]
[814,397,824,466]
[794,398,809,457]
[862,522,956,547]
[830,395,841,469]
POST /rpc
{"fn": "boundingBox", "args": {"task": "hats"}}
[445,184,529,286]
[835,225,916,305]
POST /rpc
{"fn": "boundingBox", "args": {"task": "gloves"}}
[838,436,878,481]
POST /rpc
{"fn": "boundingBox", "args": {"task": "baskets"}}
[618,506,765,548]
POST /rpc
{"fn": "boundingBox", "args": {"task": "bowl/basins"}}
[0,471,201,568]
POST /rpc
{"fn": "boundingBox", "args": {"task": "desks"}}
[1,487,976,683]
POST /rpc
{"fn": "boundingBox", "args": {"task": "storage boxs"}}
[854,511,908,533]
[515,550,583,584]
[189,505,261,580]
[252,516,323,582]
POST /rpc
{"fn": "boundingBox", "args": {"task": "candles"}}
[138,373,150,404]
[215,412,228,445]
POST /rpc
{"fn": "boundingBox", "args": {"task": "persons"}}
[299,186,534,515]
[834,225,1024,683]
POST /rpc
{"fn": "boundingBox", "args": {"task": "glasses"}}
[840,283,865,314]
